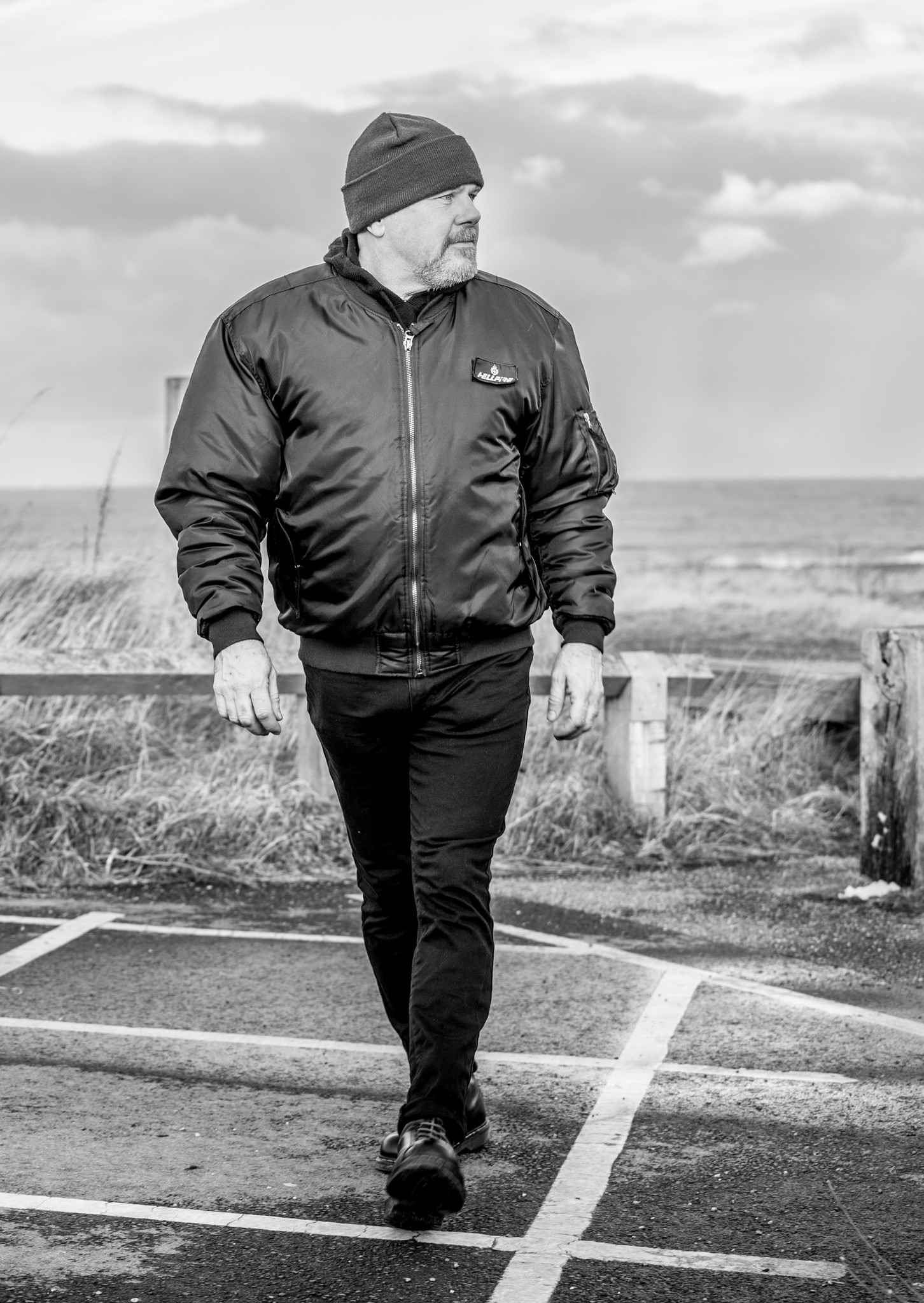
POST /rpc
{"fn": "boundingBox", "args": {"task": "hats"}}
[341,113,484,233]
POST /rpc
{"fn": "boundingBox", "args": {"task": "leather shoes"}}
[381,1115,466,1231]
[375,1073,490,1171]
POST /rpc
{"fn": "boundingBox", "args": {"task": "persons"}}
[155,111,622,1237]
